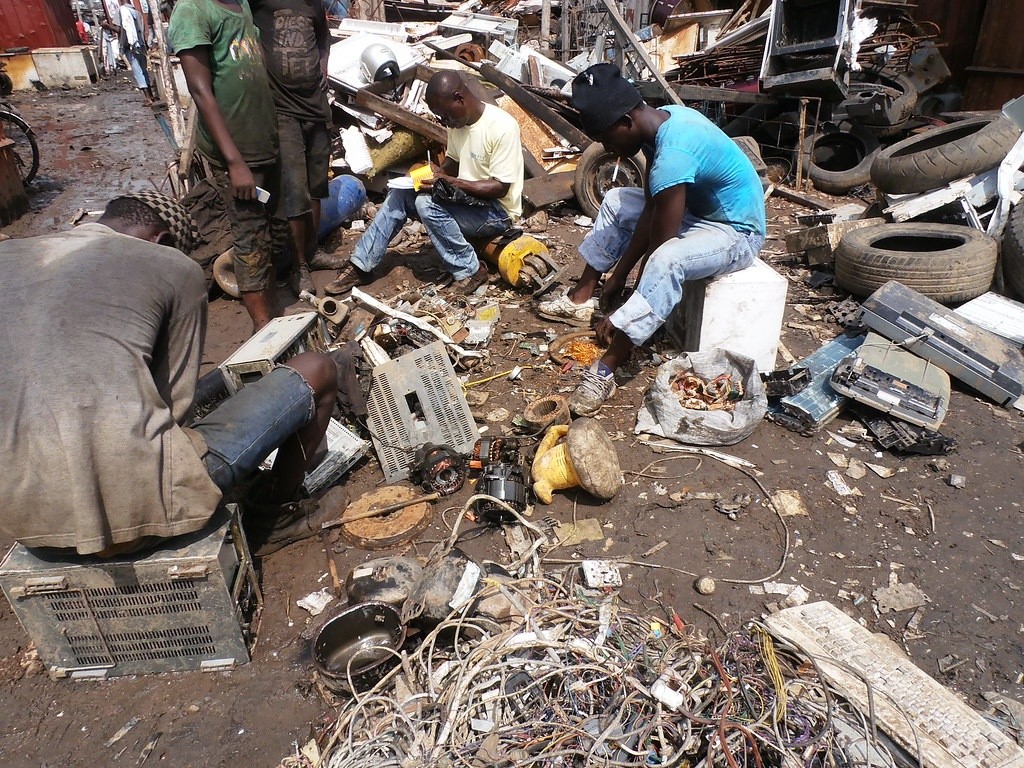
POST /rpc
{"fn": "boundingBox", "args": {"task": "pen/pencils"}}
[428,150,431,168]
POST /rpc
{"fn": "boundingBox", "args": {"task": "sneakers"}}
[247,481,350,558]
[306,248,347,270]
[538,286,595,328]
[323,259,372,295]
[566,360,619,417]
[289,263,317,296]
[455,264,489,296]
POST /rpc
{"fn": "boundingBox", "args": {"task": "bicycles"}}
[0,100,40,190]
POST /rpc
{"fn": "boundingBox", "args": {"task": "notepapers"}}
[409,164,435,193]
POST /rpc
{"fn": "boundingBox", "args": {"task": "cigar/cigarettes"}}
[611,155,621,182]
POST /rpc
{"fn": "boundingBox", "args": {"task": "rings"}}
[431,184,434,189]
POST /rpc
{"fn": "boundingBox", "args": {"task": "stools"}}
[665,253,791,380]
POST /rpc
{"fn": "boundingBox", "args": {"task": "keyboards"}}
[763,600,1024,768]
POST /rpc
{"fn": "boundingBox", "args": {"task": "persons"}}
[536,61,770,418]
[321,70,525,299]
[0,197,343,567]
[240,0,333,298]
[99,0,159,107]
[164,0,283,336]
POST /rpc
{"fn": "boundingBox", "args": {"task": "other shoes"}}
[142,97,159,107]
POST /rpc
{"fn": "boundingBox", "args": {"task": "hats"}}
[105,188,193,258]
[572,63,644,138]
